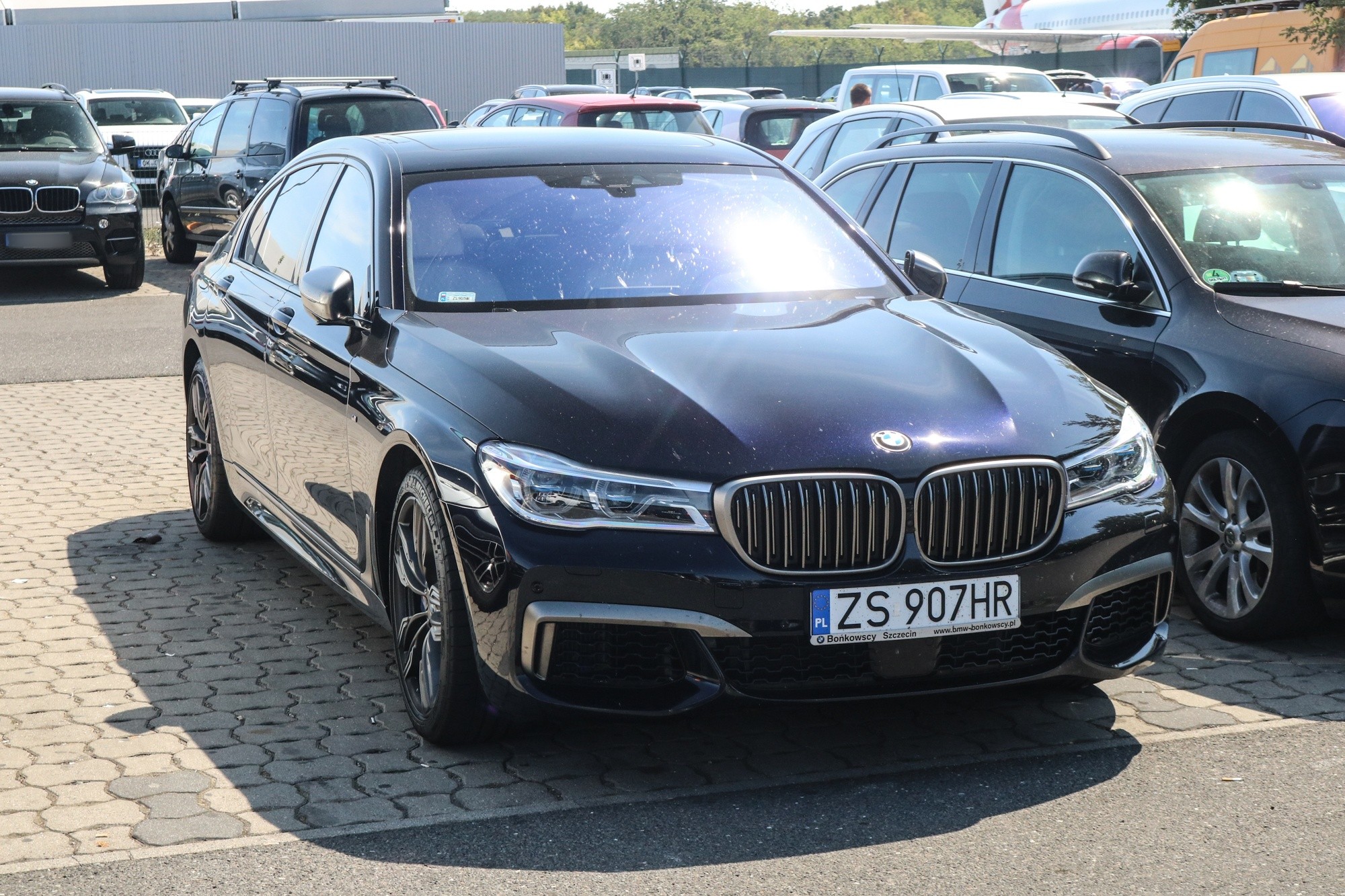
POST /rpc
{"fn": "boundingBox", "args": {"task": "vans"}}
[1161,0,1345,82]
[835,64,1061,109]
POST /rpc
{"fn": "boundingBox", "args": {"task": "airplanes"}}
[768,0,1202,64]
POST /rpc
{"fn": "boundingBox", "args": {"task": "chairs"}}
[21,106,74,147]
[309,109,351,149]
[1023,191,1110,276]
[604,121,623,129]
[906,191,974,269]
[95,108,107,121]
[411,199,507,302]
[1192,189,1281,280]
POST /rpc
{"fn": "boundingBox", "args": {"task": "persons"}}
[1070,82,1094,93]
[850,84,871,108]
[1102,84,1121,100]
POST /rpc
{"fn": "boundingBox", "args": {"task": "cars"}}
[702,99,841,161]
[456,94,716,137]
[446,99,514,129]
[782,90,1300,259]
[612,86,787,131]
[508,84,613,100]
[183,127,1182,744]
[984,69,1150,99]
[176,98,221,121]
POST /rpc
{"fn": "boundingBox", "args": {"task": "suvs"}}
[156,75,447,264]
[74,88,191,195]
[0,83,144,290]
[811,121,1344,642]
[1116,72,1345,254]
